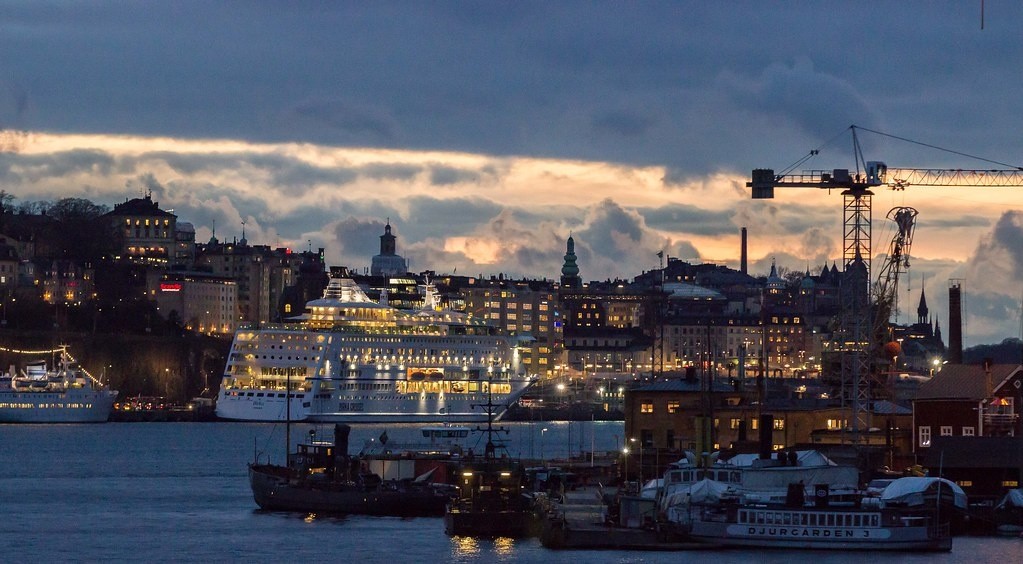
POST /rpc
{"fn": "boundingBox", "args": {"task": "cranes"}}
[873,206,920,377]
[743,123,1022,445]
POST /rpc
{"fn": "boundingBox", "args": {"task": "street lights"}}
[540,427,548,466]
[622,447,630,489]
[646,440,659,491]
[631,436,643,494]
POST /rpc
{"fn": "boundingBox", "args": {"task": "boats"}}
[47,375,63,383]
[15,379,32,389]
[0,341,119,424]
[247,367,970,553]
[430,372,445,382]
[31,380,49,388]
[410,371,426,381]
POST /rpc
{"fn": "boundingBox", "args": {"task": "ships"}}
[212,267,541,423]
